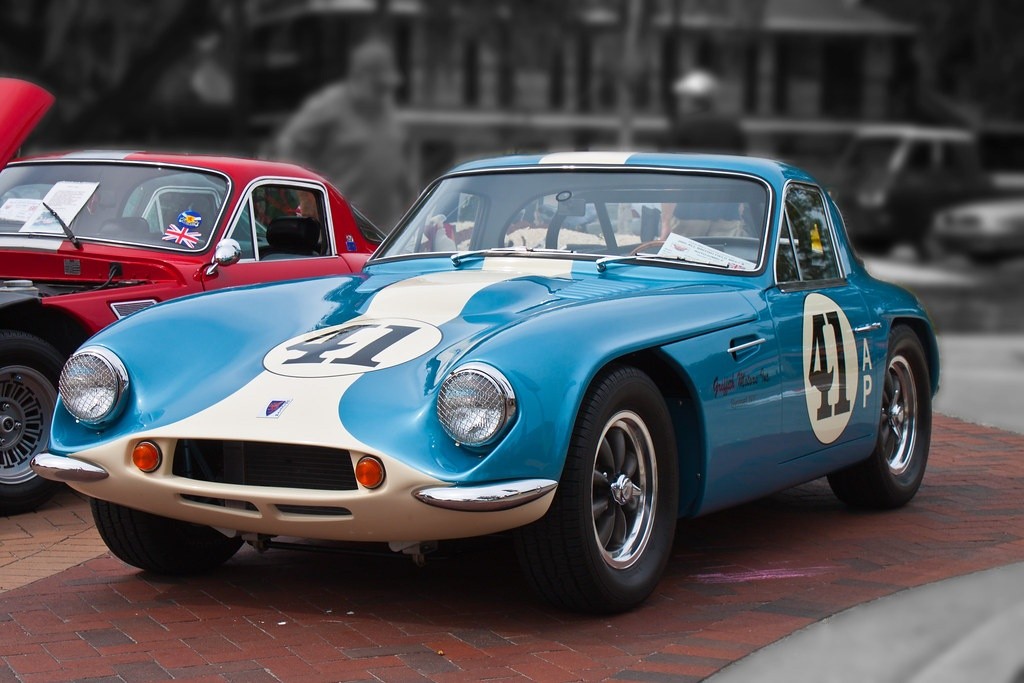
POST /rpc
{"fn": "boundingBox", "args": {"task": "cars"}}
[0,77,389,516]
[33,151,941,617]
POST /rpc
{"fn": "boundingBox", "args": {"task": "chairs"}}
[259,216,321,259]
[97,217,162,248]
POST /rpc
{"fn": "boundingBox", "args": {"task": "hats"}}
[673,72,717,99]
[350,41,402,89]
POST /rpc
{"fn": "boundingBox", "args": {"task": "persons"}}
[271,41,418,238]
[659,68,748,155]
